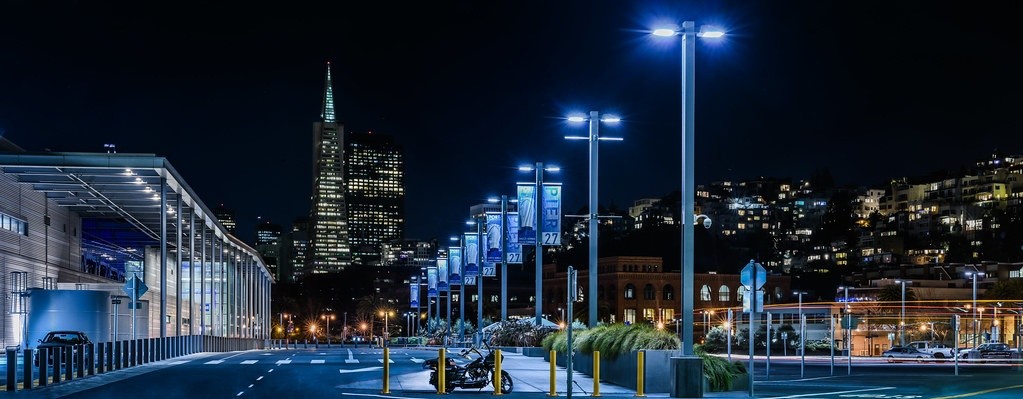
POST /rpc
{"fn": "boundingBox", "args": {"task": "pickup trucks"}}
[905,341,955,358]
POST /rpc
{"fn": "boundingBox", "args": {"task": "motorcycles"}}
[422,339,514,394]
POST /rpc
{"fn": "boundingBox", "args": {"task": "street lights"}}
[793,292,808,343]
[978,308,984,320]
[966,272,985,318]
[284,314,291,333]
[652,21,724,355]
[450,235,465,341]
[708,311,714,331]
[322,314,335,334]
[428,257,440,325]
[404,312,411,337]
[839,286,854,349]
[438,248,451,337]
[568,111,621,329]
[674,318,682,335]
[380,311,393,332]
[519,162,559,327]
[421,267,431,338]
[466,217,487,348]
[895,279,912,346]
[487,194,519,320]
[411,275,427,337]
[703,311,708,342]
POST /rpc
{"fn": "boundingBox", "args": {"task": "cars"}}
[882,347,935,363]
[34,331,94,367]
[963,343,1013,359]
